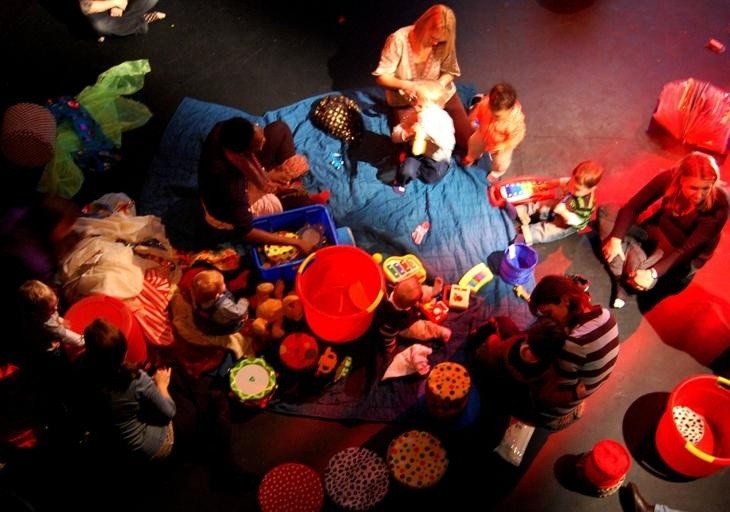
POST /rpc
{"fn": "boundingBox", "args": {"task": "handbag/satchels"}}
[309,94,363,142]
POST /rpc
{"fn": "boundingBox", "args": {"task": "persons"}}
[373,4,473,192]
[74,318,175,459]
[197,116,331,248]
[191,270,250,337]
[513,161,604,247]
[379,276,451,351]
[16,280,86,366]
[460,82,526,183]
[80,1,166,38]
[625,481,682,512]
[599,151,729,309]
[480,275,620,411]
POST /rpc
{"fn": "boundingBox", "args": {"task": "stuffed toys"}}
[252,279,303,336]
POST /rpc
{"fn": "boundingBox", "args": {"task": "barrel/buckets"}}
[654,373,730,479]
[294,245,387,343]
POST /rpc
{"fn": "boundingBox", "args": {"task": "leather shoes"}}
[626,482,649,511]
